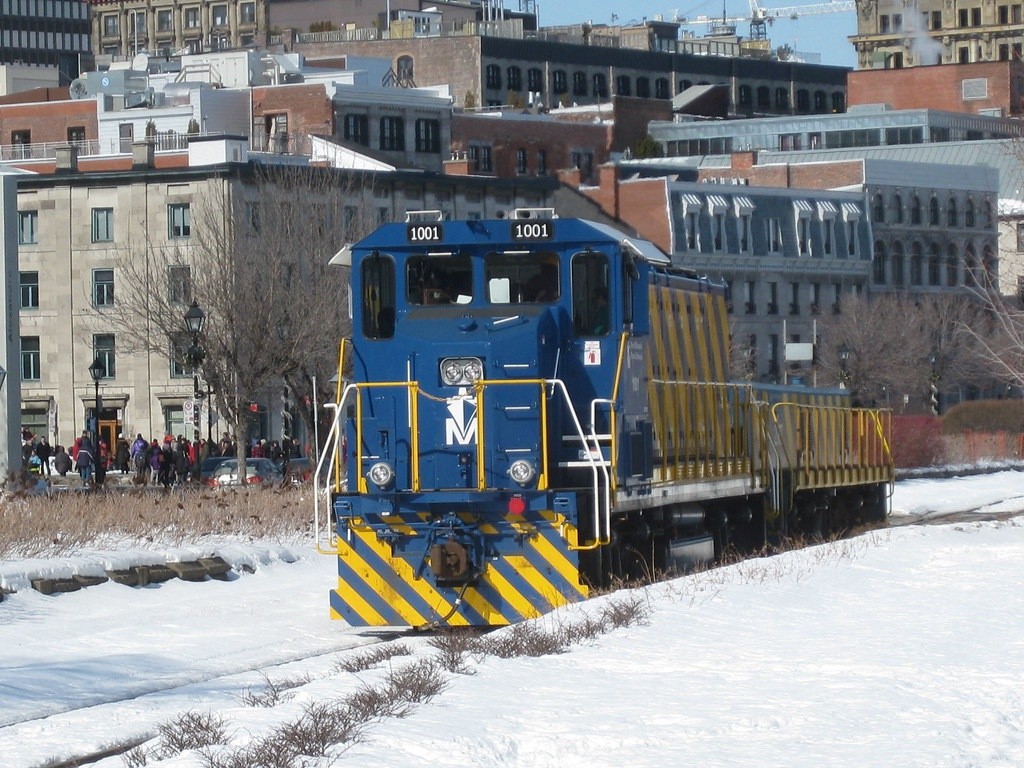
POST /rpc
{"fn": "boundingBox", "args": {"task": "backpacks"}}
[135,440,147,467]
[162,446,170,464]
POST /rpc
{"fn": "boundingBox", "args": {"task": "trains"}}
[312,208,895,646]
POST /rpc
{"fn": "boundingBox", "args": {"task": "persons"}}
[587,286,611,336]
[21,426,301,488]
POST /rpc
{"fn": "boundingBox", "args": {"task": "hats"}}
[118,433,124,438]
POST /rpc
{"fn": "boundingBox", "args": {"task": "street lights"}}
[88,357,106,484]
[184,300,206,486]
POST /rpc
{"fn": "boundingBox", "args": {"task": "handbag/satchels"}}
[158,452,165,463]
[124,450,130,460]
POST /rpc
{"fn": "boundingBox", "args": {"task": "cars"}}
[205,457,283,493]
[279,457,336,488]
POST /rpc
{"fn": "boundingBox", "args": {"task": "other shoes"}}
[80,481,90,487]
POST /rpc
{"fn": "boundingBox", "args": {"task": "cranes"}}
[672,1,857,57]
